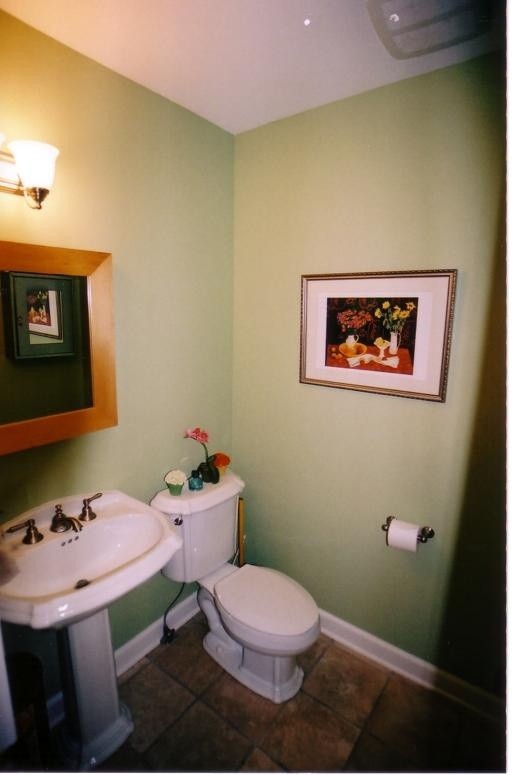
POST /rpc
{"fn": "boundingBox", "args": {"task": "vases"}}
[197,462,213,482]
[346,334,359,349]
[167,483,183,496]
[388,332,402,355]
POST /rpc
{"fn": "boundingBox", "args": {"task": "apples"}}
[331,347,343,359]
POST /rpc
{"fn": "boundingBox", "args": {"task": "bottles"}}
[189,470,203,490]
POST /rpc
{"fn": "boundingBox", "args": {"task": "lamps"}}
[0,139,62,212]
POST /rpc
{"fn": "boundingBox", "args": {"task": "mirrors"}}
[6,271,82,362]
[0,239,120,456]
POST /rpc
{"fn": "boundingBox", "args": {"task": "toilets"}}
[150,469,321,705]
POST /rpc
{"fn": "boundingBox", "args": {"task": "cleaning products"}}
[186,471,202,490]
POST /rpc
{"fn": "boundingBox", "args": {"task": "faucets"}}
[51,504,83,535]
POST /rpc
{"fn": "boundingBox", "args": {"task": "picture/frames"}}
[28,290,63,340]
[299,267,458,403]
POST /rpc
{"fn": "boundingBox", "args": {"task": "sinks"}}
[0,507,183,630]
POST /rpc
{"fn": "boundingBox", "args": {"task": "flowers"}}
[335,300,417,336]
[185,427,222,483]
[164,469,188,486]
